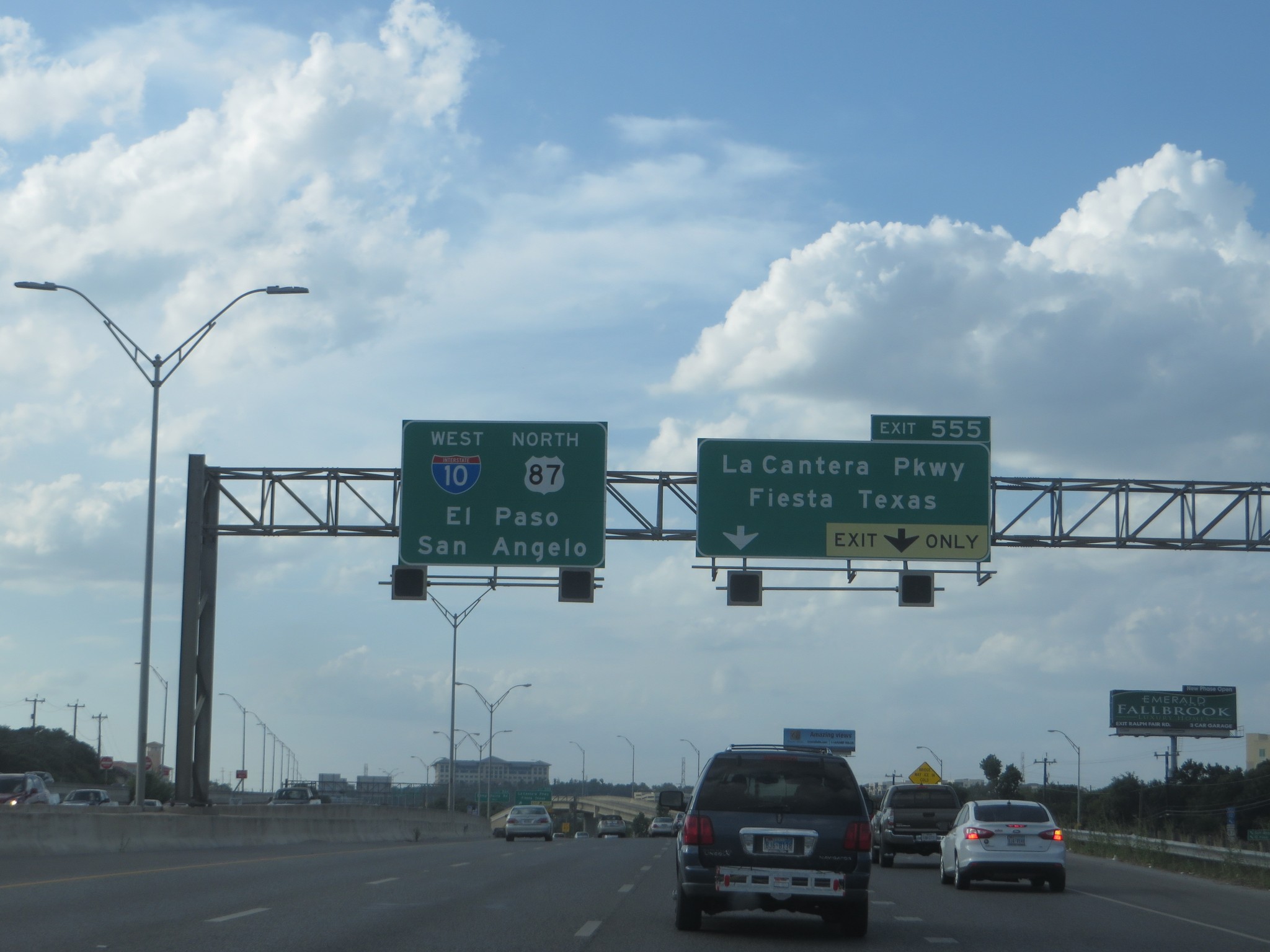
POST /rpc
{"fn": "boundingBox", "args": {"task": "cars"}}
[574,831,590,839]
[646,811,686,836]
[598,814,627,838]
[0,770,175,808]
[490,805,565,842]
[938,799,1067,893]
[270,787,323,805]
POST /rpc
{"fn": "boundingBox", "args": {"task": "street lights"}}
[218,691,300,804]
[1048,728,1081,828]
[134,660,169,772]
[916,746,943,784]
[12,277,309,809]
[432,728,512,815]
[411,755,448,810]
[680,738,700,777]
[378,767,403,779]
[617,735,635,798]
[569,740,585,796]
[454,680,532,819]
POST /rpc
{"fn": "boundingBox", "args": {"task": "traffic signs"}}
[400,418,605,568]
[696,436,994,564]
[513,790,552,806]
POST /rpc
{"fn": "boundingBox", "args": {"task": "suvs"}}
[874,783,964,867]
[674,744,873,936]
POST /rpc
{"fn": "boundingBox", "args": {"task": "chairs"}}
[711,772,762,804]
[911,797,927,808]
[791,782,825,803]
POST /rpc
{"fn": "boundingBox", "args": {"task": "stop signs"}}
[145,756,152,771]
[100,757,114,769]
